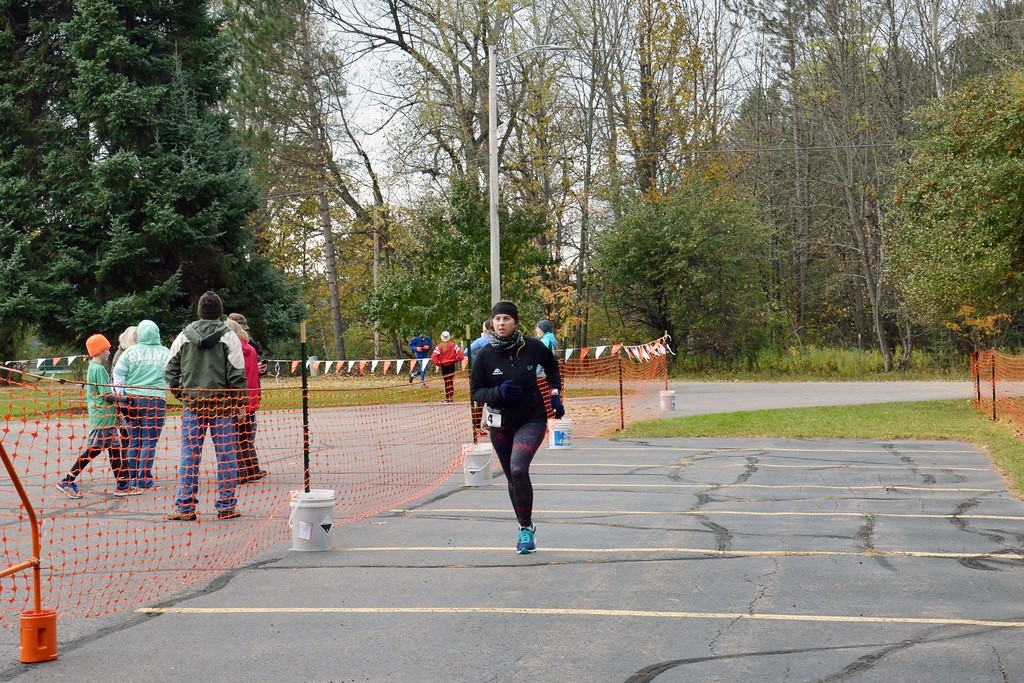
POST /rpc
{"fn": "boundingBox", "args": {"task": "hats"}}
[197,292,222,319]
[86,335,110,359]
[440,331,451,342]
[229,314,249,331]
[538,320,552,334]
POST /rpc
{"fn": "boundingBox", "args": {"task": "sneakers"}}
[516,524,536,554]
[167,509,196,520]
[219,509,240,518]
[114,485,145,496]
[55,479,83,499]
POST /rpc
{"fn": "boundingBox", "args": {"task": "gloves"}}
[550,393,565,418]
[498,379,522,399]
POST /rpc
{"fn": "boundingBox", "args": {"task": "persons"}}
[532,319,558,378]
[472,301,566,554]
[109,326,139,479]
[56,331,143,499]
[409,332,433,383]
[113,319,173,491]
[431,331,464,404]
[463,318,496,437]
[488,414,492,422]
[223,312,267,485]
[165,290,248,521]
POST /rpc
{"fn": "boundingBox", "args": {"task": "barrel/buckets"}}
[461,442,494,486]
[660,391,676,411]
[288,489,336,552]
[548,419,573,449]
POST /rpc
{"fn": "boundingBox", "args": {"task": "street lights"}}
[487,46,572,311]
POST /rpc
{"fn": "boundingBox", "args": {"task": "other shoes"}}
[237,470,267,483]
